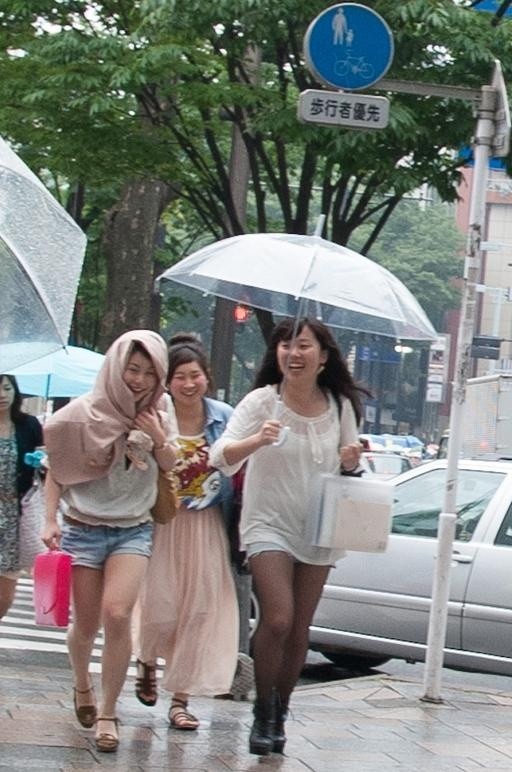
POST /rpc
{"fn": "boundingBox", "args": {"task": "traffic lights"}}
[234,302,248,334]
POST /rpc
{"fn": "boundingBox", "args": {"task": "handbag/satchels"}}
[308,452,396,556]
[149,465,178,527]
[33,544,73,628]
[15,465,52,575]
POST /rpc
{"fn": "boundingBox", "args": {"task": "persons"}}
[37,329,184,753]
[206,316,363,754]
[133,330,240,730]
[0,374,46,619]
[459,530,472,541]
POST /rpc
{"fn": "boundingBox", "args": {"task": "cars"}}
[350,429,441,481]
[250,458,511,679]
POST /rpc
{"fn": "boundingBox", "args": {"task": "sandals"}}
[168,697,201,731]
[95,715,121,753]
[135,658,158,706]
[71,686,98,728]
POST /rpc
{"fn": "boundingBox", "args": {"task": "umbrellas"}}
[0,135,89,374]
[155,210,438,450]
[1,341,106,399]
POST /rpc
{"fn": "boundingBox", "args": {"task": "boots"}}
[248,697,276,756]
[269,699,289,754]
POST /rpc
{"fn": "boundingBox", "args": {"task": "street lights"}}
[395,344,414,431]
